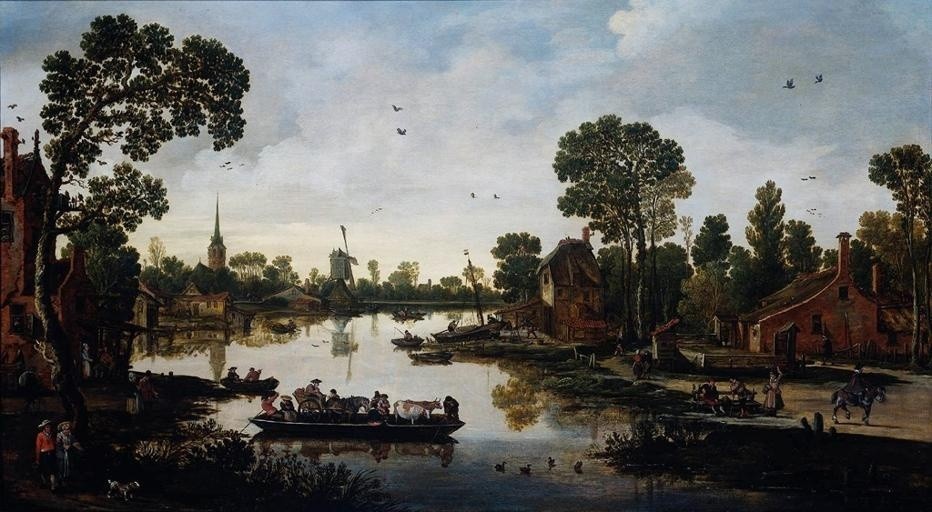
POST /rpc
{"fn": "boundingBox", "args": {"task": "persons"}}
[0,319,874,493]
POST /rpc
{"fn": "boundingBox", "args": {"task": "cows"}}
[406,397,442,410]
[394,400,432,424]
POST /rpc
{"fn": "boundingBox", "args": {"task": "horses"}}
[325,397,370,423]
[831,383,886,426]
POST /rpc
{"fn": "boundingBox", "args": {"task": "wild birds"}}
[397,128,406,135]
[8,104,17,109]
[17,117,24,122]
[64,175,85,202]
[783,78,795,89]
[815,74,822,84]
[97,160,107,165]
[392,105,402,111]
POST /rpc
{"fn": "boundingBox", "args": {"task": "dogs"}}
[105,479,141,502]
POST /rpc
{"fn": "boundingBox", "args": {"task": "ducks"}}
[495,461,507,470]
[574,459,583,474]
[520,464,532,473]
[548,457,557,471]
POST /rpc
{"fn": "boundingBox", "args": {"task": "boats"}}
[330,305,380,316]
[430,324,478,343]
[247,412,465,436]
[391,310,426,319]
[271,324,296,333]
[220,376,279,391]
[390,335,425,347]
[408,351,455,362]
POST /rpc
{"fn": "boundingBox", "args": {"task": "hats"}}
[281,395,291,401]
[38,419,50,427]
[228,367,237,370]
[311,379,321,383]
